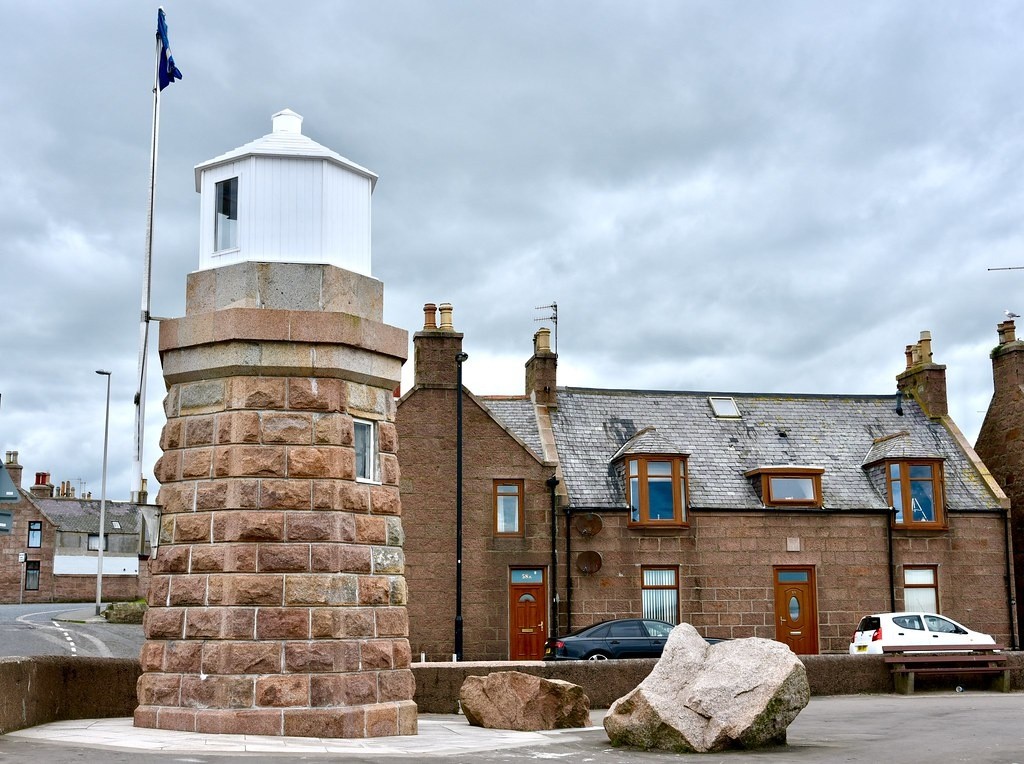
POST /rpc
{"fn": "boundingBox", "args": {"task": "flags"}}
[156,10,182,91]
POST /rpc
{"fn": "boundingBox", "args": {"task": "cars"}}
[849,612,1000,654]
[544,618,734,661]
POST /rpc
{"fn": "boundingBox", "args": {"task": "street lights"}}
[454,352,469,662]
[95,369,112,616]
